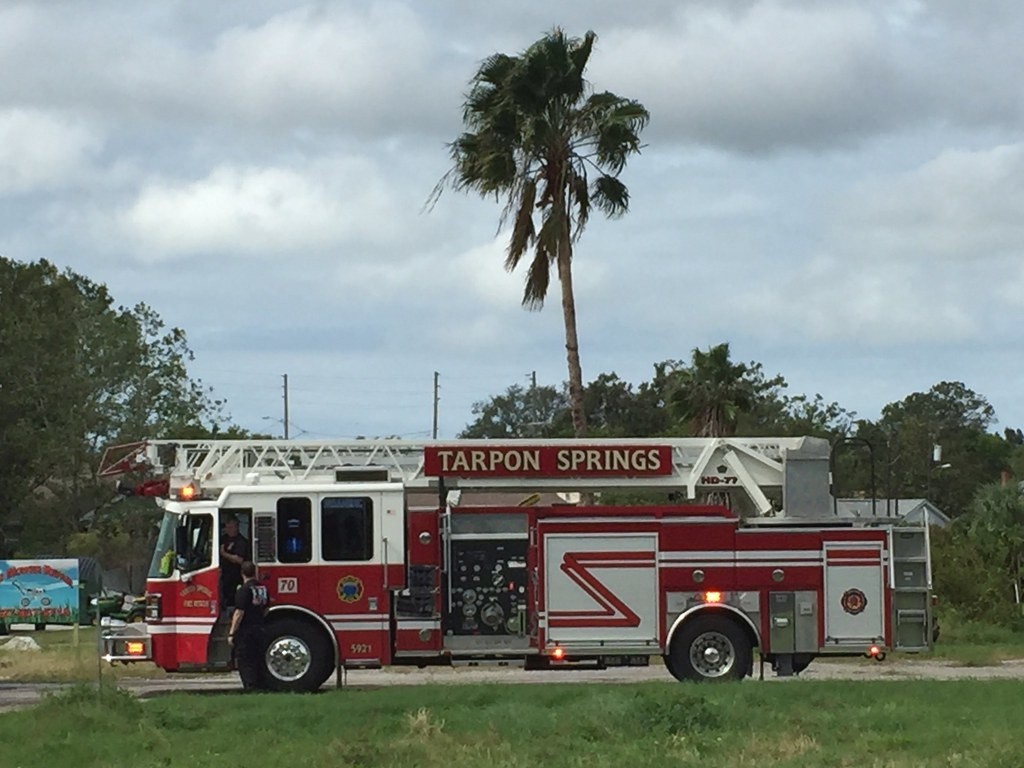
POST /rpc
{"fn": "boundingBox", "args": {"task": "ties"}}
[293,537,296,553]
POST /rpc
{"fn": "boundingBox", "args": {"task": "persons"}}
[218,516,272,694]
[925,617,940,643]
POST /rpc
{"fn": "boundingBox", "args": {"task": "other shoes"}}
[242,684,255,693]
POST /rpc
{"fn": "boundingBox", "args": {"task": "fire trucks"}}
[94,435,944,696]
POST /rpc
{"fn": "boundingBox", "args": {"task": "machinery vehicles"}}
[88,591,147,626]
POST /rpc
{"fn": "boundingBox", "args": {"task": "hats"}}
[288,519,300,528]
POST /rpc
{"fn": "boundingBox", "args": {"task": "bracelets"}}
[228,635,234,637]
